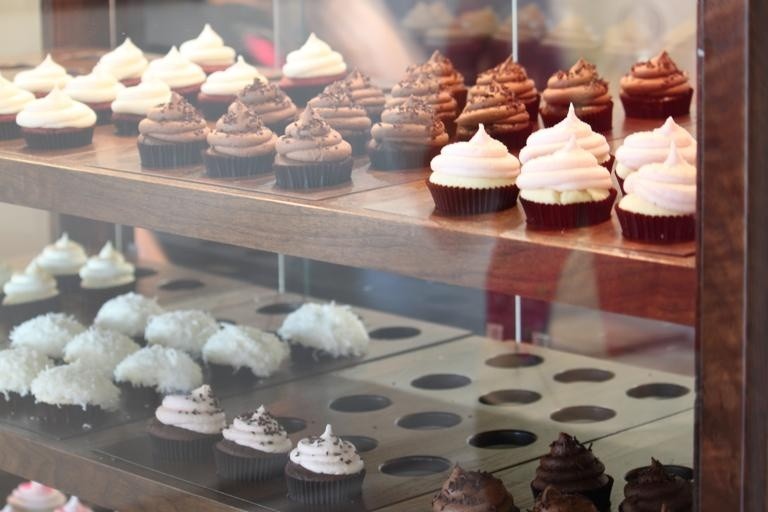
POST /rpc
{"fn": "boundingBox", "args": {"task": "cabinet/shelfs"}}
[0,0,767,512]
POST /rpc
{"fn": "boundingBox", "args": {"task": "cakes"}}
[519,99,621,170]
[54,491,94,512]
[420,122,521,214]
[512,134,610,229]
[611,142,698,242]
[212,405,297,486]
[150,384,227,470]
[617,454,695,511]
[36,233,89,295]
[285,423,368,506]
[612,117,696,194]
[5,480,68,512]
[79,240,138,308]
[1,260,66,326]
[528,433,615,510]
[433,463,522,511]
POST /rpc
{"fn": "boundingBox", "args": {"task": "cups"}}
[36,400,103,432]
[209,360,270,386]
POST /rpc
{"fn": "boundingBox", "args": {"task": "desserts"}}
[137,50,694,191]
[1,24,349,150]
[2,290,370,434]
